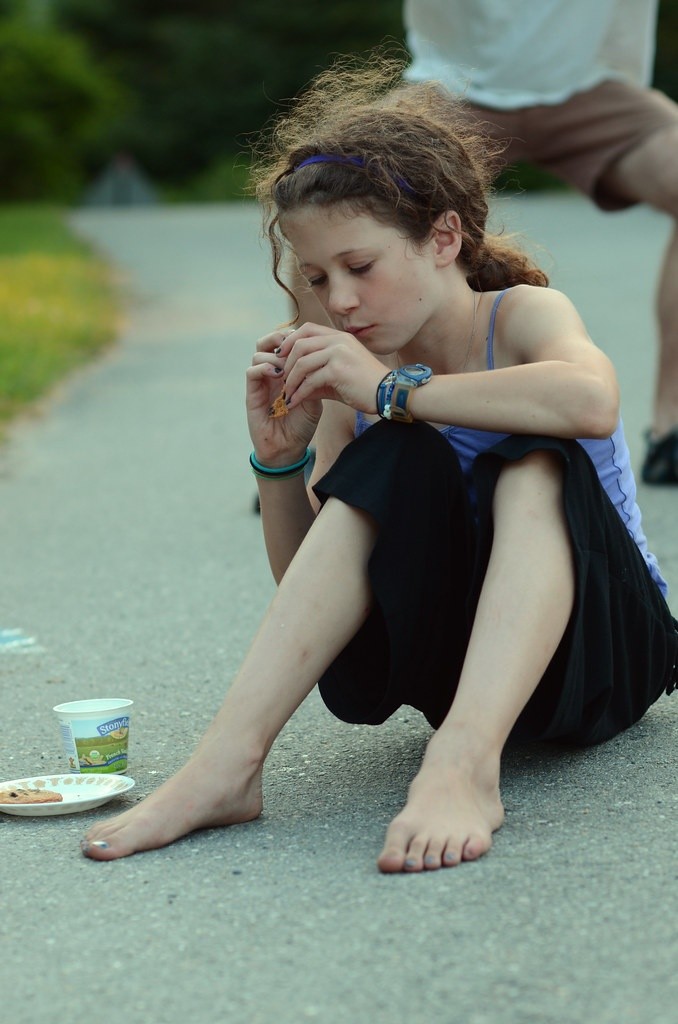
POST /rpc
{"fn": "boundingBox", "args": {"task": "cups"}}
[53,698,134,776]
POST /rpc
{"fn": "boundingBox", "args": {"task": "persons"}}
[82,106,678,877]
[392,0,678,486]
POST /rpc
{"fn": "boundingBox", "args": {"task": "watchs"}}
[390,363,433,423]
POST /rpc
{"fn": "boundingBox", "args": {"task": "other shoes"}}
[641,424,678,485]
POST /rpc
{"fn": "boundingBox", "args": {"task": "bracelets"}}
[249,446,311,480]
[376,369,398,419]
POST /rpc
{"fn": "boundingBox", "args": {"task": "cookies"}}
[0,789,63,805]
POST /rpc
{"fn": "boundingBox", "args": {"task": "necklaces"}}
[395,291,476,437]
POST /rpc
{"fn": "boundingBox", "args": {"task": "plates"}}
[0,773,136,817]
